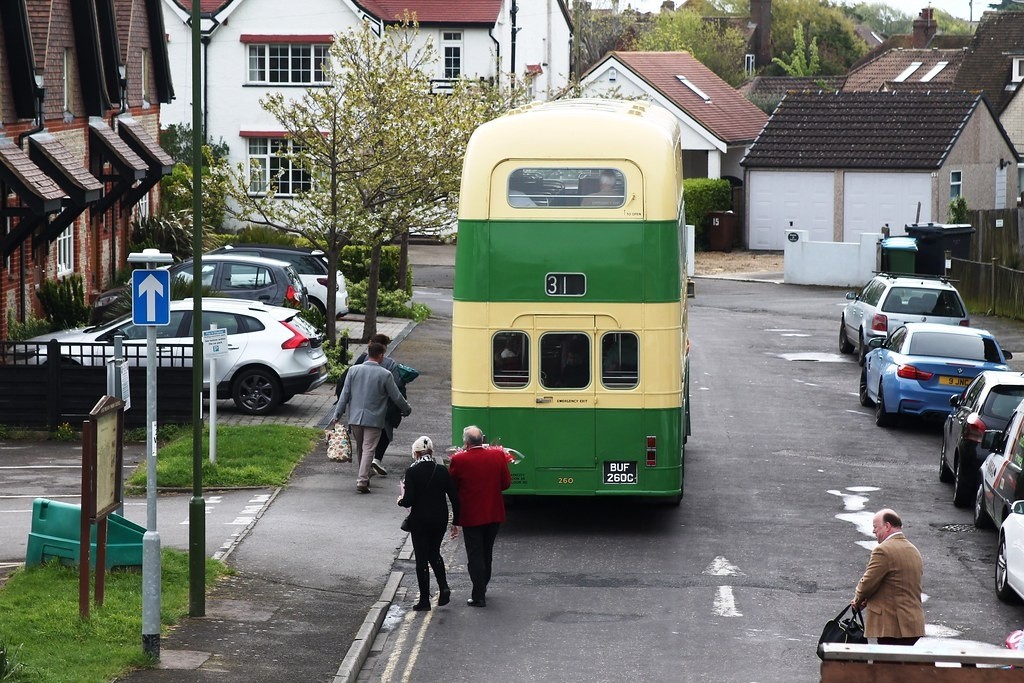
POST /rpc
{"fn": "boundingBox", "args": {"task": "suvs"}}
[6,296,330,416]
[128,245,349,325]
[90,254,309,325]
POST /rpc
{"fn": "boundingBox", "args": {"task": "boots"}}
[413,568,431,611]
[430,558,451,606]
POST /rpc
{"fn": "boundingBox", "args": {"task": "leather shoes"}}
[467,598,485,607]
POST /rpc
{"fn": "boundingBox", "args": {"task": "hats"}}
[411,436,433,458]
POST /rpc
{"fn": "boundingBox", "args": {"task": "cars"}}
[974,398,1024,531]
[995,499,1024,603]
[938,371,1024,506]
[860,322,1014,429]
[837,270,970,366]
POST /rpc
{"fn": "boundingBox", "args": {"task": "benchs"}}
[817,642,1024,683]
[601,370,637,389]
[494,369,547,388]
[508,180,625,208]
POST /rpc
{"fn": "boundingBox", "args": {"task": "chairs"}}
[886,294,903,312]
[922,293,940,313]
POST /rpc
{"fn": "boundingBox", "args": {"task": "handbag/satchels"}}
[816,603,869,661]
[324,422,352,463]
[396,363,419,383]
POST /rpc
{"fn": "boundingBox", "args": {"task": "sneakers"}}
[371,459,388,475]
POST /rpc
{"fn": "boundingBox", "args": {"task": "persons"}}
[450,426,511,607]
[509,177,537,207]
[333,342,412,493]
[359,334,406,476]
[851,509,926,645]
[580,170,623,206]
[398,436,459,612]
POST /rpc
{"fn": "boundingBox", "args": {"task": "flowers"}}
[446,437,526,465]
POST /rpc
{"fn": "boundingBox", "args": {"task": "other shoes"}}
[356,485,371,494]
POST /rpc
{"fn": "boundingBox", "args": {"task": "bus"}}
[450,96,695,507]
